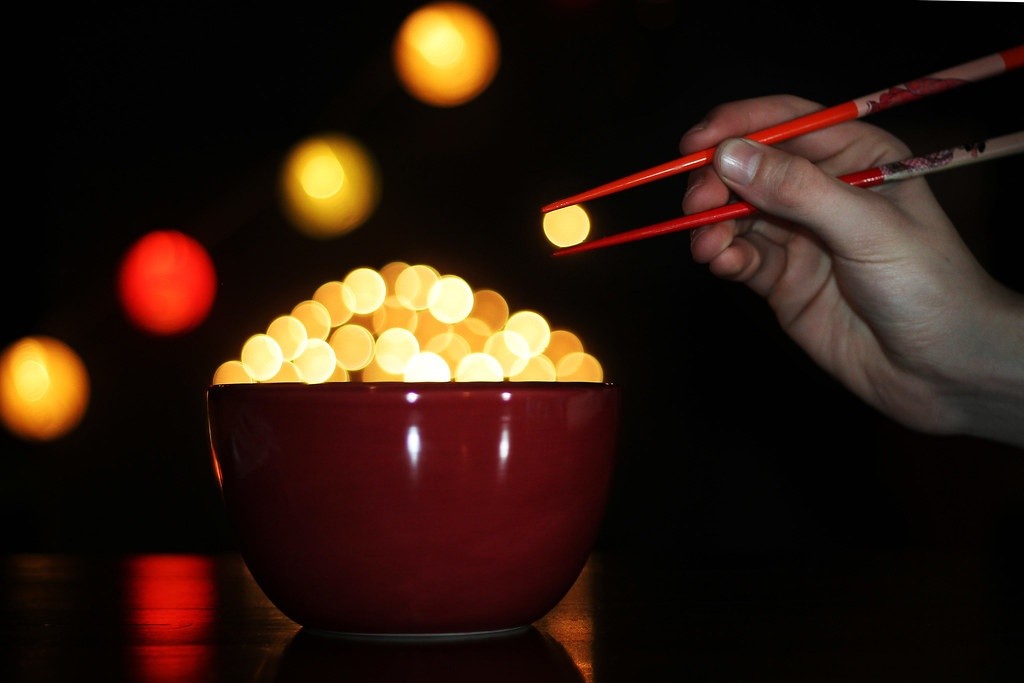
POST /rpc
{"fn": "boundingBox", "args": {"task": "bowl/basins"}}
[207,381,622,636]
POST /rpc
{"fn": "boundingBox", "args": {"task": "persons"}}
[681,94,1024,444]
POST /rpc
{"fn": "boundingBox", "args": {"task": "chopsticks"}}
[541,48,1024,257]
